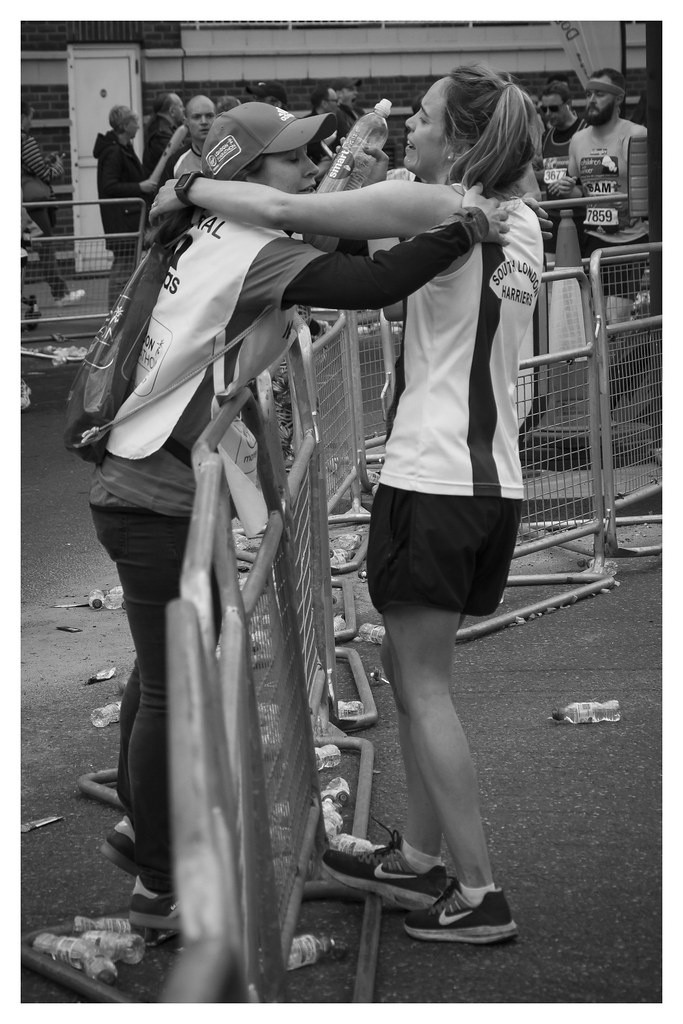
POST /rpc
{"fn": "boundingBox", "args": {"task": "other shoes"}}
[21,378,32,410]
[55,289,86,308]
[100,808,141,877]
[129,874,182,930]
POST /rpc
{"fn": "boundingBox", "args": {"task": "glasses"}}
[540,100,567,113]
[326,98,338,103]
[252,95,267,101]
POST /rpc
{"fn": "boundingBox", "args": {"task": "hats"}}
[245,81,288,104]
[201,102,338,181]
[333,77,362,92]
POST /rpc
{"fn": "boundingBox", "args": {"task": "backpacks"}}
[64,204,195,470]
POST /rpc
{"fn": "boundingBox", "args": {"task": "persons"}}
[149,66,553,947]
[87,99,509,931]
[22,68,649,413]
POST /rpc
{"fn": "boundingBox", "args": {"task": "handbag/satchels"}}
[21,134,50,203]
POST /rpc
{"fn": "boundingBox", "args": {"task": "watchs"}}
[175,171,206,210]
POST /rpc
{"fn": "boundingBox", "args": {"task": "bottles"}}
[587,558,618,577]
[91,700,123,727]
[366,468,381,496]
[315,743,341,771]
[250,613,273,668]
[259,934,336,971]
[358,622,389,647]
[337,699,365,719]
[31,917,145,981]
[319,777,386,855]
[302,98,392,253]
[105,586,125,611]
[88,590,104,609]
[232,532,248,549]
[551,699,621,723]
[329,533,362,567]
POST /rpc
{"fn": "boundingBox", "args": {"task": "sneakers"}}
[403,876,519,944]
[322,816,447,912]
